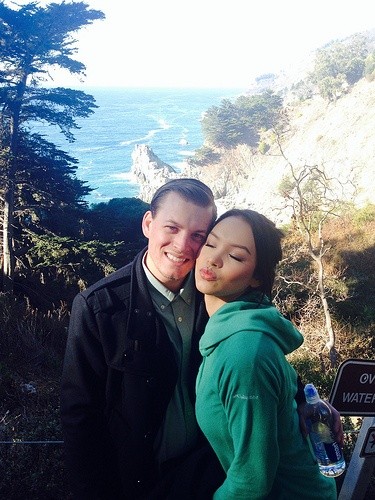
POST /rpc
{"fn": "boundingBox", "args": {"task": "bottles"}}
[302,383,345,478]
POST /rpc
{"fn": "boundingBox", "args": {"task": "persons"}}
[60,178,345,500]
[195,210,338,500]
